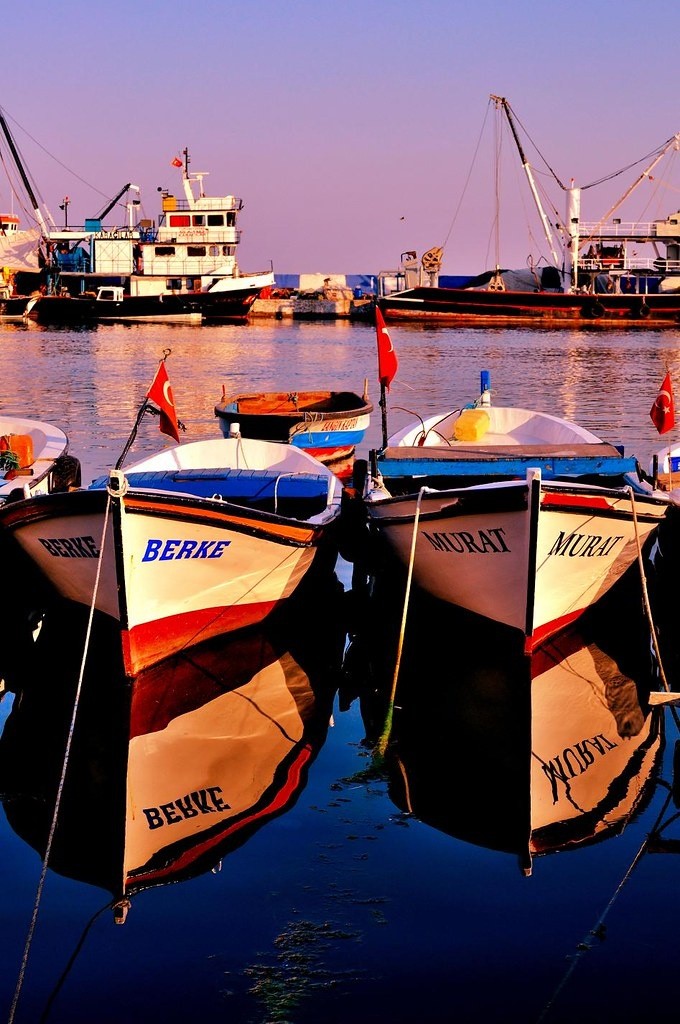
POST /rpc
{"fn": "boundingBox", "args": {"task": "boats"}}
[1,420,348,676]
[207,372,373,467]
[377,95,678,326]
[342,575,669,875]
[0,408,75,511]
[3,571,342,921]
[355,371,674,652]
[0,102,276,321]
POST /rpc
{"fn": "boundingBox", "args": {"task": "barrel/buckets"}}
[0,436,34,479]
[454,409,490,442]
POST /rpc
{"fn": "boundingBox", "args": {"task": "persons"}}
[602,281,614,294]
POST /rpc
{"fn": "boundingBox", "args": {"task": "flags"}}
[375,304,398,393]
[147,363,180,443]
[649,373,675,435]
